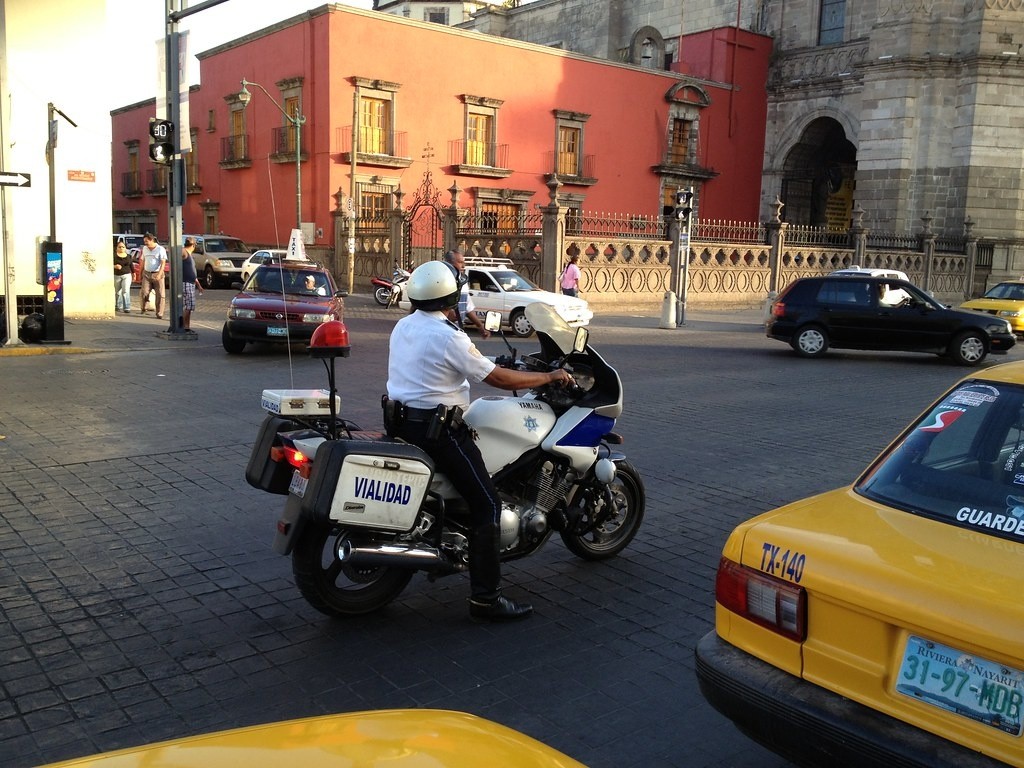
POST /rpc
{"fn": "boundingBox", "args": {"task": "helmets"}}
[406,260,463,312]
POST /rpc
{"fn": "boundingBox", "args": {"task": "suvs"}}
[182,234,258,290]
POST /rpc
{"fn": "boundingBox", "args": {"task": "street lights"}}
[239,77,306,229]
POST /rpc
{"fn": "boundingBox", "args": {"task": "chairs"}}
[265,275,284,290]
[856,290,868,303]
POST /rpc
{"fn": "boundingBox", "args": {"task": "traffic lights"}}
[148,117,175,166]
[674,190,693,221]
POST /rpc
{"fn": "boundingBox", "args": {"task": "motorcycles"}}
[246,301,648,619]
[371,260,415,310]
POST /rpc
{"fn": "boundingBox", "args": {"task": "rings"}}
[567,374,572,379]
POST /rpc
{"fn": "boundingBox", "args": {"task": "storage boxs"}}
[300,439,432,533]
[260,389,342,416]
[245,415,363,498]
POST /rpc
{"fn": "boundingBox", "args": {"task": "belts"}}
[400,409,435,421]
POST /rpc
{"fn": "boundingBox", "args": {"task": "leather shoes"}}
[476,595,534,624]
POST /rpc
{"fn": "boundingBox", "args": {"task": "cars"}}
[128,248,170,289]
[399,257,594,338]
[959,276,1024,336]
[26,709,591,768]
[113,234,160,258]
[765,277,1017,367]
[241,250,317,288]
[816,265,912,304]
[692,361,1023,768]
[222,229,348,359]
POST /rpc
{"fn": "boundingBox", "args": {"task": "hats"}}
[186,237,197,246]
[306,275,315,281]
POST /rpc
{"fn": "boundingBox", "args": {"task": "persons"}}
[879,286,910,307]
[447,248,490,339]
[562,255,581,298]
[305,275,326,295]
[183,236,203,335]
[138,234,168,319]
[381,260,575,619]
[114,242,136,313]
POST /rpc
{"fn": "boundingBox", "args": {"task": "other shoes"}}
[185,328,195,332]
[156,313,162,319]
[124,310,130,313]
[141,309,147,315]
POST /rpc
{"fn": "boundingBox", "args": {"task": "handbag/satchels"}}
[557,268,565,284]
